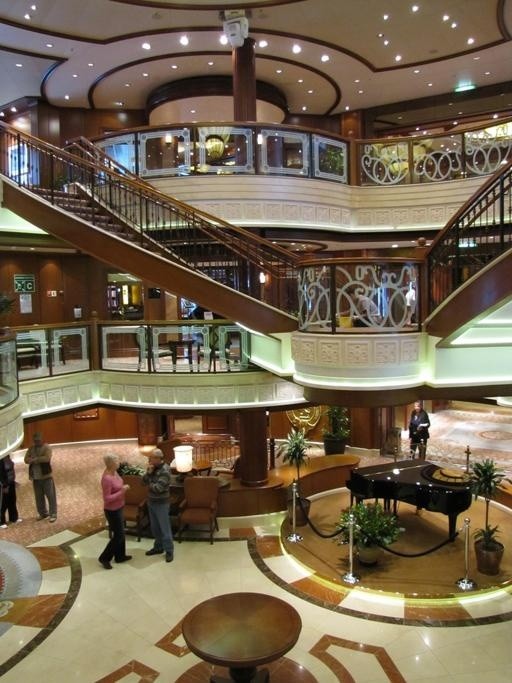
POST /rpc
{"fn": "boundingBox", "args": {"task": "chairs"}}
[34,335,66,368]
[134,326,174,372]
[16,347,39,369]
[176,475,219,545]
[105,474,151,541]
[197,330,233,372]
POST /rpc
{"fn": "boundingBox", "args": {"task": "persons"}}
[337,286,381,326]
[406,402,430,457]
[1,457,24,529]
[98,453,134,570]
[25,432,59,523]
[142,449,177,562]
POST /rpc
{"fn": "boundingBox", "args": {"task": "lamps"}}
[198,122,231,158]
[173,444,194,484]
[370,135,432,175]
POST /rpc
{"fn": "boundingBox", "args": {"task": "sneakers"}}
[165,553,174,562]
[0,514,58,529]
[145,547,163,555]
[96,556,113,571]
[114,554,132,563]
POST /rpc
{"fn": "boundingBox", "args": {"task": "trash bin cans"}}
[287,498,310,527]
[387,427,402,455]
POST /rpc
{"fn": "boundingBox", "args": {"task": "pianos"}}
[346,459,473,543]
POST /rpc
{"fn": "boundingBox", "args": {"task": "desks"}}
[143,494,189,535]
[181,591,302,683]
[168,339,201,373]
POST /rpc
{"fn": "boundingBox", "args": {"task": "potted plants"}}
[333,501,406,563]
[465,457,512,576]
[320,406,351,454]
[276,426,311,527]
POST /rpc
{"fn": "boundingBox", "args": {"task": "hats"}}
[138,446,165,458]
[33,432,43,441]
